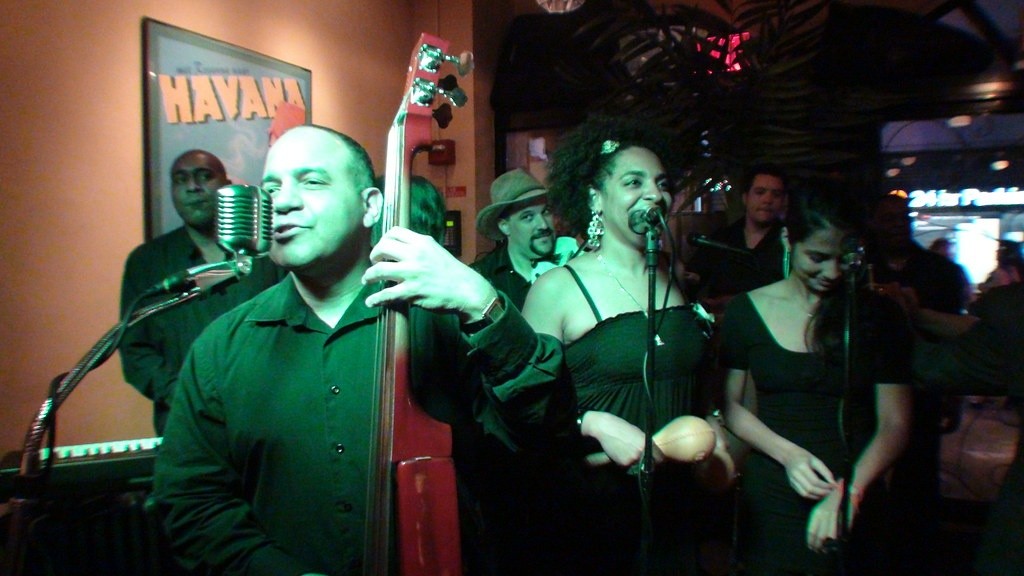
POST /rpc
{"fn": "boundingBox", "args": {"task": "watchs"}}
[460,290,506,335]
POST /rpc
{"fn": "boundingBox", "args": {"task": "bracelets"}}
[846,486,862,499]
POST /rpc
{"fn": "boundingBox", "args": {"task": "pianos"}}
[0,434,164,498]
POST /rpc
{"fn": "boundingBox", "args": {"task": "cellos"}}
[361,32,475,576]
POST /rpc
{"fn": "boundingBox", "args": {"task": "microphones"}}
[215,184,274,260]
[628,205,661,235]
[687,232,754,255]
[839,235,864,267]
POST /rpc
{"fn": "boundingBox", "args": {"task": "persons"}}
[932,239,1024,338]
[119,150,290,437]
[522,140,734,576]
[453,169,578,311]
[863,196,970,519]
[679,161,793,312]
[154,124,579,576]
[370,176,462,576]
[718,179,915,576]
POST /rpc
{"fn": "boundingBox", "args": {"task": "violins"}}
[528,234,716,324]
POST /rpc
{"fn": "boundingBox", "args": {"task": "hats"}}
[475,169,550,241]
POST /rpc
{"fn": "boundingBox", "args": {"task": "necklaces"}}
[593,248,666,345]
[786,277,813,316]
